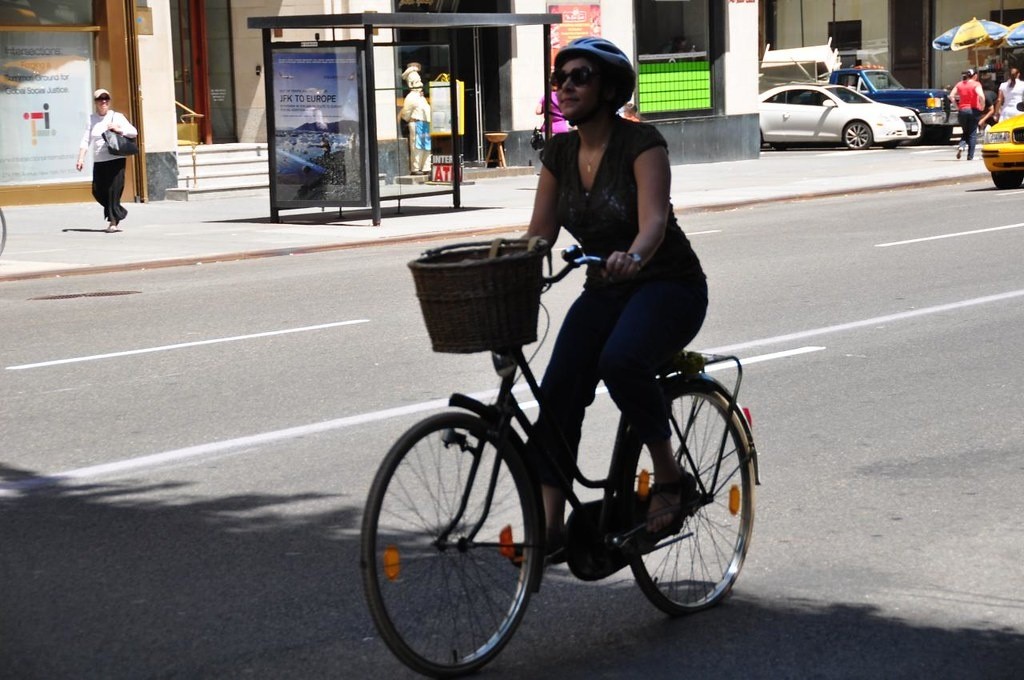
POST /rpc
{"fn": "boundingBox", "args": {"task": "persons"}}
[949,68,986,160]
[401,65,433,174]
[301,87,329,131]
[76,88,138,232]
[978,90,998,131]
[511,37,708,568]
[992,68,1024,122]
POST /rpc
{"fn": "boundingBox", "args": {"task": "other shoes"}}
[956,147,962,160]
[106,218,118,233]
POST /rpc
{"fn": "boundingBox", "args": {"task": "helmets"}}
[554,37,634,111]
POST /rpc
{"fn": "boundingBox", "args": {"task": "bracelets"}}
[78,160,85,162]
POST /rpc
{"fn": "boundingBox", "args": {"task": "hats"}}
[94,88,110,100]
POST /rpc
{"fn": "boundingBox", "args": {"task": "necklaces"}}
[582,143,605,174]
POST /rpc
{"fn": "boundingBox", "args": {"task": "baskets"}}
[407,238,546,354]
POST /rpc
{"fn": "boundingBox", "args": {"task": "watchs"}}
[627,252,642,270]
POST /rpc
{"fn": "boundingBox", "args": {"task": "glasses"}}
[548,65,601,86]
[96,96,109,100]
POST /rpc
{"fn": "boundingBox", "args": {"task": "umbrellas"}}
[989,20,1024,49]
[931,16,1009,73]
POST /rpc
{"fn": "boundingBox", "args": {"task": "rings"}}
[617,258,624,263]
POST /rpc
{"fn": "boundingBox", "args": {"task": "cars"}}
[757,84,922,150]
[981,102,1024,190]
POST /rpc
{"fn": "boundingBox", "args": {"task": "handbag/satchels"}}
[102,111,138,157]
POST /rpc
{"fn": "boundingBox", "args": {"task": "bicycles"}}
[359,237,762,680]
[0,212,8,257]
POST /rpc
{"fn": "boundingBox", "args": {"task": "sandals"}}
[640,464,696,539]
[511,526,568,569]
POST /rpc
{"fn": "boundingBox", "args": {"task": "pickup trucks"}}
[828,66,961,144]
